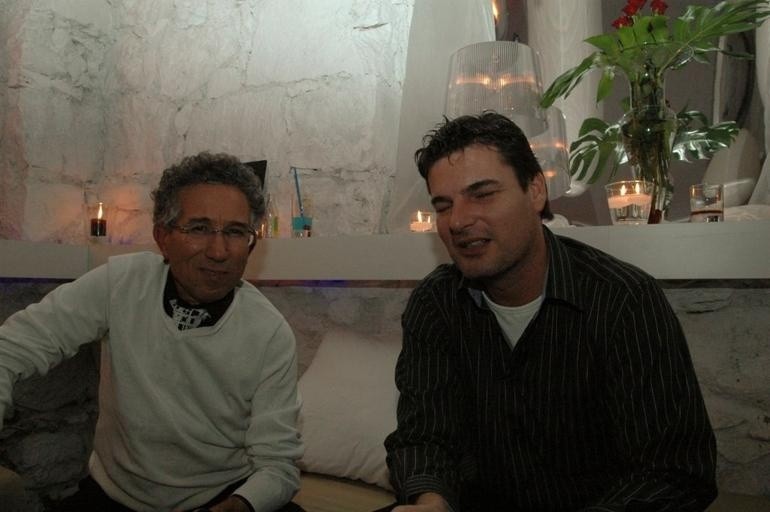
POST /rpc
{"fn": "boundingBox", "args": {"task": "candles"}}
[611,184,651,219]
[91,202,106,236]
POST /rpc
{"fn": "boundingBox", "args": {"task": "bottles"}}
[252,190,278,238]
[90,200,106,237]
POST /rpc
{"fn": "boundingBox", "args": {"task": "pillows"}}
[299,327,401,493]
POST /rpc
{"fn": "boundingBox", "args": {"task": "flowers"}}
[541,0,770,183]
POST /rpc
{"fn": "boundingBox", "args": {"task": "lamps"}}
[444,41,547,141]
[529,103,570,202]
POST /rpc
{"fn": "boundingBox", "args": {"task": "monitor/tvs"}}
[237,159,269,189]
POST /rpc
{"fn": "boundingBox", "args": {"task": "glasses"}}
[163,222,257,242]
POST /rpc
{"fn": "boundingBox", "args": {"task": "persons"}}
[374,107,719,512]
[0,150,307,512]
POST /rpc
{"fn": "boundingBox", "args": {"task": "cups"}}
[686,182,726,224]
[600,180,656,225]
[290,193,314,240]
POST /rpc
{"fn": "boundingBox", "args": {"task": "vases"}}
[619,71,676,222]
